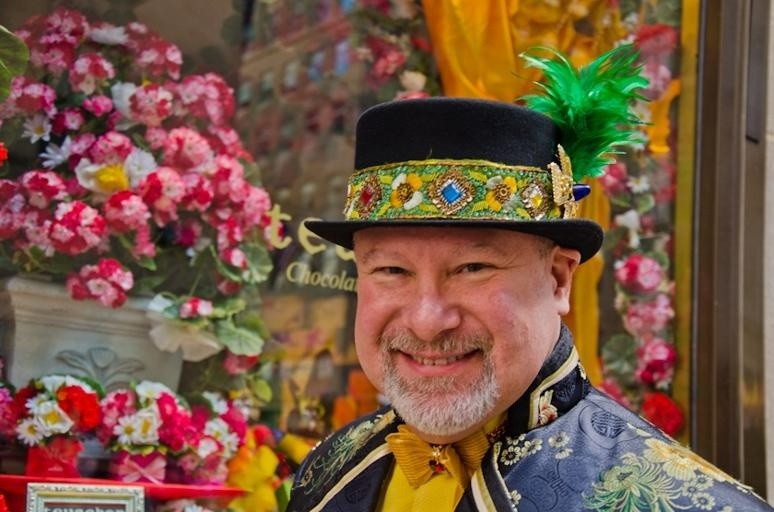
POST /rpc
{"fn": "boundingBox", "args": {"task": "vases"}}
[10,276,191,400]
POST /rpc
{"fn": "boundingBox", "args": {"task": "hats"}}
[304,96,610,267]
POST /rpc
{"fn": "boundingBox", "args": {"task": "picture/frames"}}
[27,483,145,512]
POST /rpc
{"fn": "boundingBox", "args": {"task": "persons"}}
[285,96,774,512]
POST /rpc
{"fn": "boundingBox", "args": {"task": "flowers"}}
[353,0,447,97]
[0,0,319,399]
[593,5,685,435]
[1,372,316,512]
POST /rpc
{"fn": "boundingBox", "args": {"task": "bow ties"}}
[382,421,495,493]
